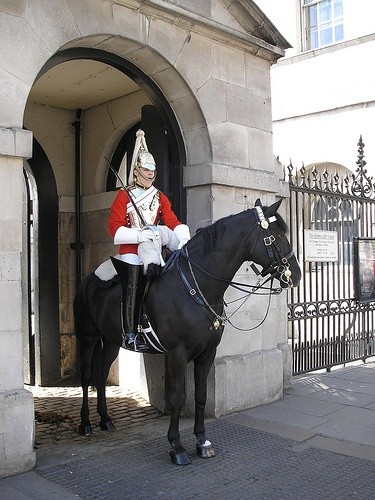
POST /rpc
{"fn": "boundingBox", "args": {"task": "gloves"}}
[113,225,155,245]
[172,223,192,250]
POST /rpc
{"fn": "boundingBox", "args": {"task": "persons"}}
[109,128,190,350]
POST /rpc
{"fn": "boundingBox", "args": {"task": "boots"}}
[109,255,150,352]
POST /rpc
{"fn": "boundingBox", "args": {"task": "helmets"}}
[127,128,157,188]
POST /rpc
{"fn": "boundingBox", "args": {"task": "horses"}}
[72,195,302,466]
